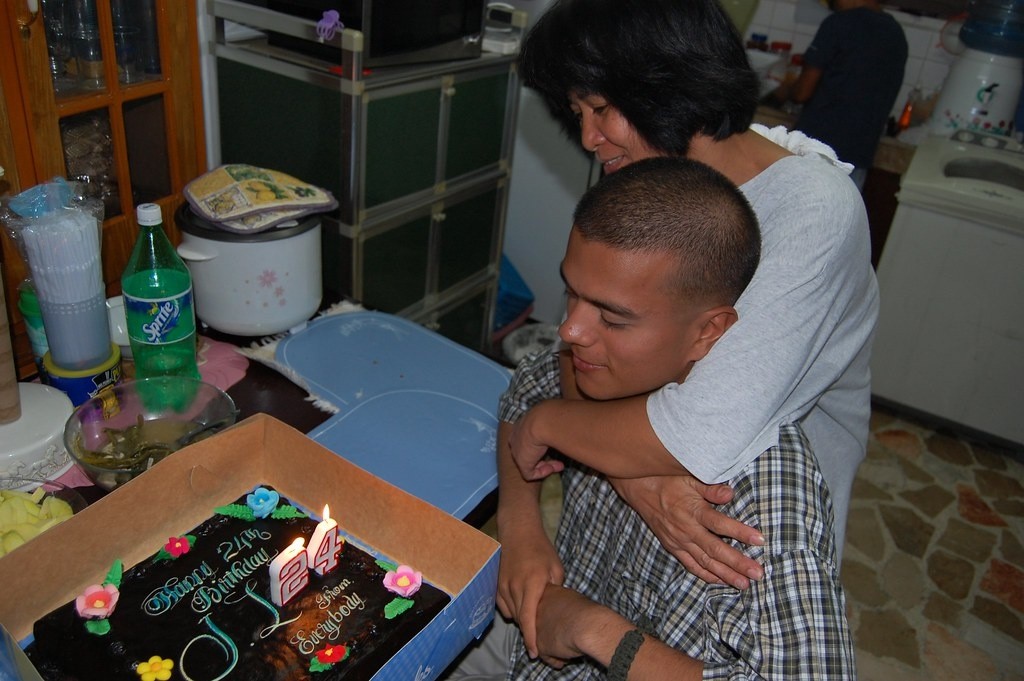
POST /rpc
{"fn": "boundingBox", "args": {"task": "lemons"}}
[248,181,276,203]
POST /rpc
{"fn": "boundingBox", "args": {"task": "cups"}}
[45,343,122,408]
[771,41,791,62]
[41,0,162,91]
[39,284,111,370]
[18,286,49,359]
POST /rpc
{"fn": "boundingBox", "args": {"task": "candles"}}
[269,504,343,607]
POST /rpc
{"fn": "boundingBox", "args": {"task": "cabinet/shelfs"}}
[209,33,523,360]
[499,83,605,331]
[1,0,209,382]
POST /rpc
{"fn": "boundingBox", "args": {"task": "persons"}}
[788,1,909,194]
[436,156,853,681]
[509,1,880,589]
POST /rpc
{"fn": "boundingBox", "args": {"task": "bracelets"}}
[608,616,655,681]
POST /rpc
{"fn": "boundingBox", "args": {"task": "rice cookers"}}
[174,202,329,336]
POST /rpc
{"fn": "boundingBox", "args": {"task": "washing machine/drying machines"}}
[871,128,1024,461]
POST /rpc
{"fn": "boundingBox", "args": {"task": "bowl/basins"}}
[0,479,85,556]
[63,376,236,487]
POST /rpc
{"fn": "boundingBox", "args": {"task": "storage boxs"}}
[0,412,502,681]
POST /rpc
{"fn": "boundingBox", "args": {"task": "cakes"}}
[15,483,455,681]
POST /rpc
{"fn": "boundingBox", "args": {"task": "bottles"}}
[898,85,922,126]
[959,0,1024,56]
[746,33,769,53]
[122,204,202,411]
